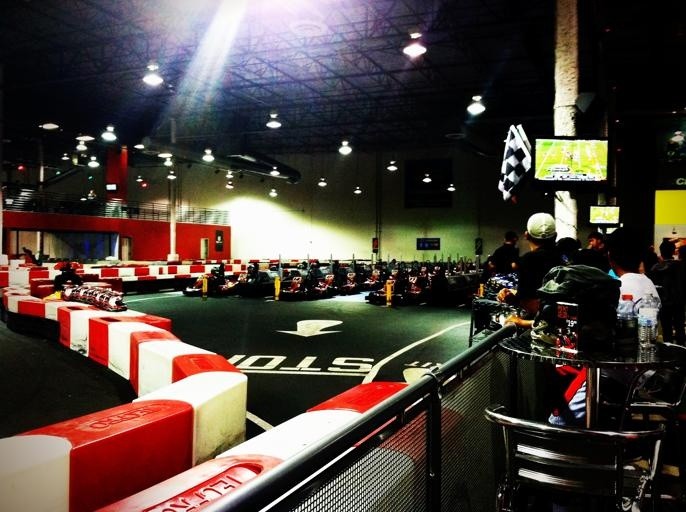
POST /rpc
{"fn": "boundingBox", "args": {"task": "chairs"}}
[623,360,686,511]
[482,403,664,511]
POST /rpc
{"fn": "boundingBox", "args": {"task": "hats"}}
[525,211,558,240]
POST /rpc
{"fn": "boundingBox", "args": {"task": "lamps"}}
[403,2,486,116]
[40,61,287,196]
[316,135,465,203]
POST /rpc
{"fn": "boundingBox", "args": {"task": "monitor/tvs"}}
[532,136,613,186]
[589,205,621,226]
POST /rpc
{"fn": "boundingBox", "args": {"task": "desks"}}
[497,336,684,429]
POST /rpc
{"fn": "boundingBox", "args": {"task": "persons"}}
[24,249,35,263]
[54,265,83,292]
[483,212,686,426]
[189,258,479,300]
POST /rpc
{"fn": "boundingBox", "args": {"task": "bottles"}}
[638,292,660,349]
[615,294,636,318]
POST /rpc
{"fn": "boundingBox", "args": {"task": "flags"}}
[499,122,532,201]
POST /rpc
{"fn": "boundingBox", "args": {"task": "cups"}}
[613,315,638,337]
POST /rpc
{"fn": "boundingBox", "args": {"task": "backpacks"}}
[531,265,625,343]
